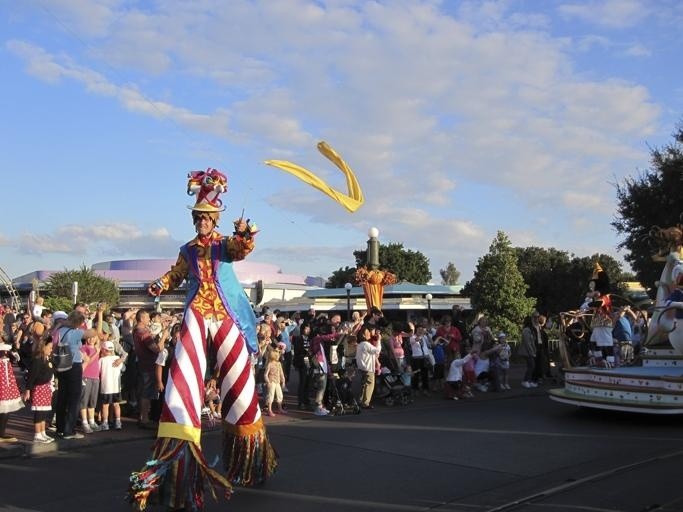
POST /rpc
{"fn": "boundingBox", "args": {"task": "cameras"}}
[96,303,103,308]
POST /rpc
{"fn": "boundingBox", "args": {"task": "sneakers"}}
[0,418,122,444]
[263,383,510,416]
[529,382,537,387]
[521,381,530,388]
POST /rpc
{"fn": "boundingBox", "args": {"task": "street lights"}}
[354,227,397,316]
[344,283,353,320]
[71,281,79,305]
[425,294,433,327]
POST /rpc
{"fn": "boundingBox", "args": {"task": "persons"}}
[521,292,648,388]
[253,306,512,417]
[0,289,222,444]
[123,212,278,512]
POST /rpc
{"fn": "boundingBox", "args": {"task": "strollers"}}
[201,402,216,430]
[376,370,412,408]
[324,367,361,416]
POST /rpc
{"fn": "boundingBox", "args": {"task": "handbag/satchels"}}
[53,341,72,373]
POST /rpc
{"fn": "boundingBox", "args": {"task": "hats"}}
[187,168,228,213]
[102,341,115,351]
[96,321,110,334]
